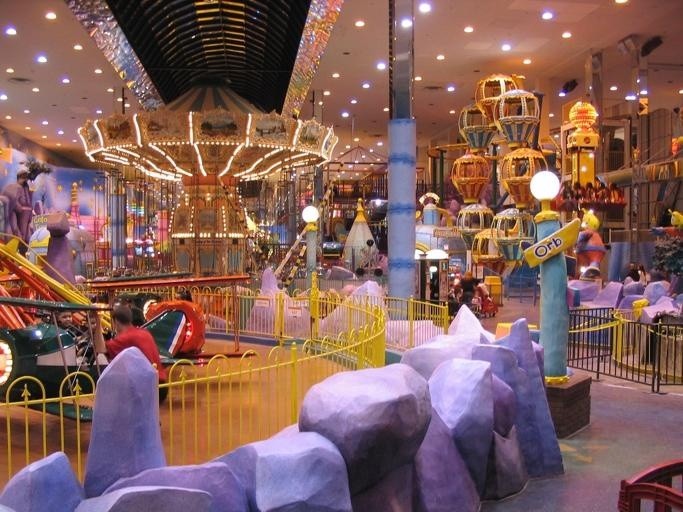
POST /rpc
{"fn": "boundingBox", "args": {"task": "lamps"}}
[530,170,563,223]
[301,205,320,232]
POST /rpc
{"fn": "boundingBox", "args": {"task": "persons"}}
[636,264,647,286]
[559,178,623,204]
[650,263,683,296]
[76,311,108,351]
[173,291,192,300]
[93,305,169,401]
[624,263,641,285]
[50,308,83,339]
[459,271,477,301]
[477,279,489,312]
